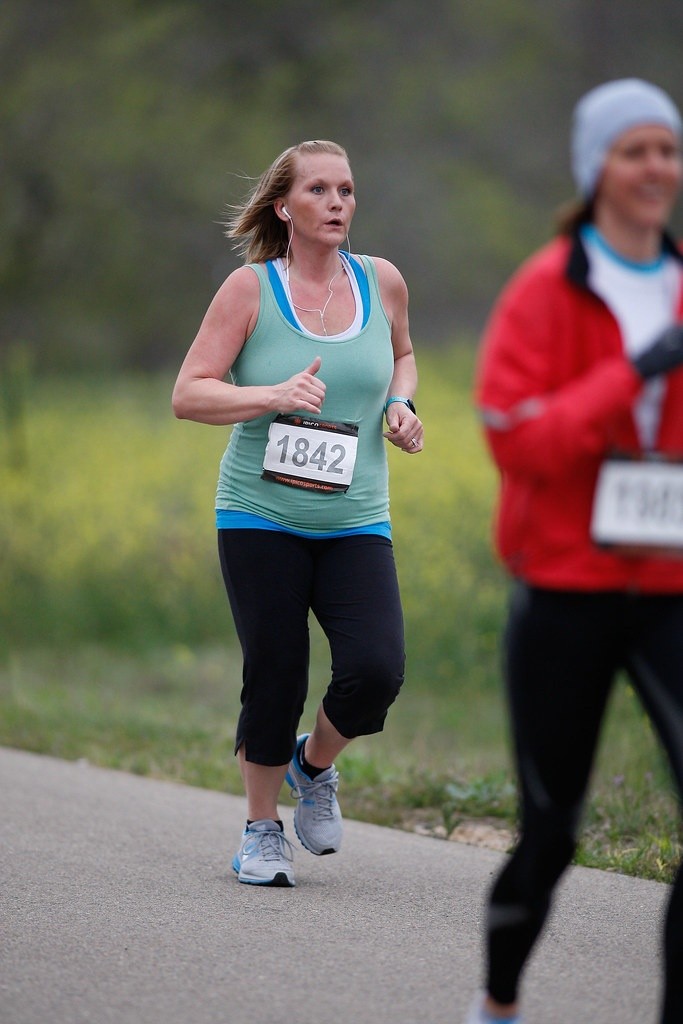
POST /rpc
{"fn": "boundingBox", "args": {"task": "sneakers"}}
[232,817,295,887]
[285,732,344,855]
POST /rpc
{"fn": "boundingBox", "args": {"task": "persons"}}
[171,138,425,886]
[478,82,683,1024]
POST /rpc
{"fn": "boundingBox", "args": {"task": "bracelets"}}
[385,397,416,415]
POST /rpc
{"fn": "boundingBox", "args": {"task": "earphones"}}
[281,206,292,219]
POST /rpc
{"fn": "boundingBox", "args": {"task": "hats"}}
[569,79,681,203]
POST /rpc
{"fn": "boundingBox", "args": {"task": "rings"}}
[412,438,419,447]
